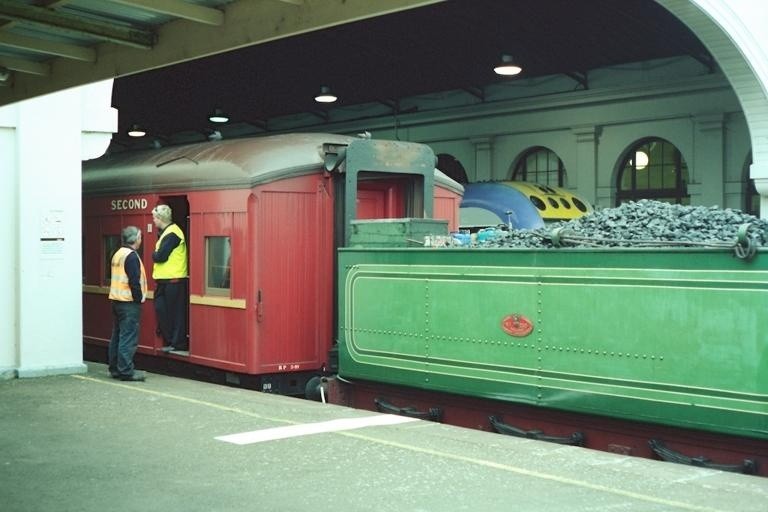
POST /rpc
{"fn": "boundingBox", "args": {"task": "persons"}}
[108,226,145,381]
[152,204,189,352]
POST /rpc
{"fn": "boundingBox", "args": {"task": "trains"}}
[454,179,593,234]
[79,130,766,473]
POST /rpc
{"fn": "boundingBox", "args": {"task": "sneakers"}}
[110,369,147,381]
[160,343,188,352]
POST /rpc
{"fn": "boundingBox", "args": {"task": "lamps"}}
[312,84,340,107]
[205,106,231,124]
[125,121,148,139]
[492,54,523,78]
